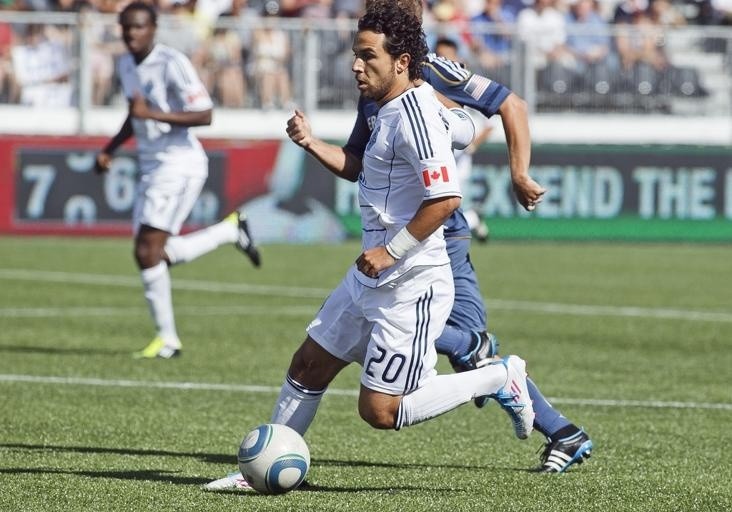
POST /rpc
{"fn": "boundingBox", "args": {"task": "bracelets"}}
[387,226,420,262]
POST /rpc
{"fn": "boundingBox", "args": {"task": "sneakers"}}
[456,328,498,409]
[541,430,593,473]
[133,337,183,356]
[205,471,252,489]
[227,210,260,266]
[495,354,535,438]
[466,212,489,241]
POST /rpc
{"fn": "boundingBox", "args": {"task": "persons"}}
[202,2,538,495]
[285,2,592,478]
[433,33,494,246]
[94,1,260,359]
[1,0,732,114]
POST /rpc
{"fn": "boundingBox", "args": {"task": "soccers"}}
[237,423,311,494]
